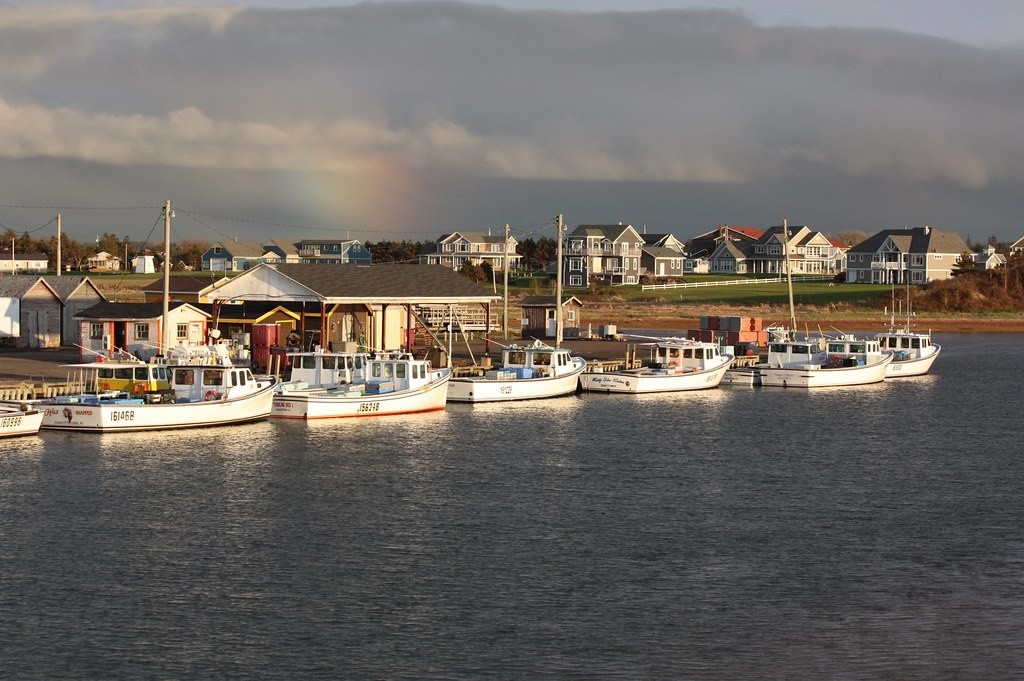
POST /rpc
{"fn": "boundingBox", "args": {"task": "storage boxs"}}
[500,367,532,378]
[354,380,394,395]
[894,351,917,361]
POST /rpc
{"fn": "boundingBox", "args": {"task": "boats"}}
[0,404,45,438]
[11,355,277,433]
[578,337,735,394]
[444,341,586,404]
[272,347,453,418]
[753,334,893,388]
[870,273,941,381]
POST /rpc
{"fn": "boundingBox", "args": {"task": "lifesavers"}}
[536,367,550,378]
[205,390,219,401]
[330,323,337,334]
[831,355,842,367]
[101,383,111,390]
[288,333,300,347]
[134,383,148,394]
[670,361,679,366]
